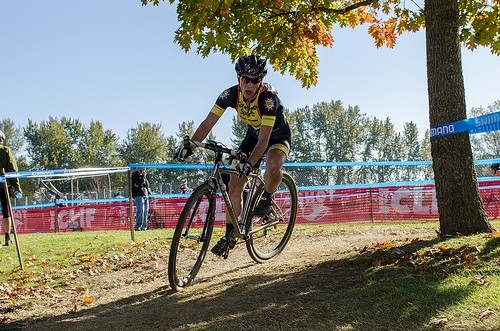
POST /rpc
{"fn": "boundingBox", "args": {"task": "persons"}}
[491,163,500,176]
[114,192,127,203]
[132,162,152,230]
[0,131,22,246]
[181,185,190,193]
[172,54,292,255]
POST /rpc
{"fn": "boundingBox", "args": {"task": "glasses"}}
[240,76,261,84]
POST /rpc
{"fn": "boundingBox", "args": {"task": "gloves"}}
[236,160,254,178]
[177,135,197,161]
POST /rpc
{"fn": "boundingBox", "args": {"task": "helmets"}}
[0,130,6,140]
[235,54,267,79]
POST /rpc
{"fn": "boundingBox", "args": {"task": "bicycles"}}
[168,135,297,292]
[482,189,500,221]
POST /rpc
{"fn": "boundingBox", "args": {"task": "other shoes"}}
[5,240,12,245]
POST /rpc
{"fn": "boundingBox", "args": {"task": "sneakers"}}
[254,196,274,216]
[211,237,236,256]
